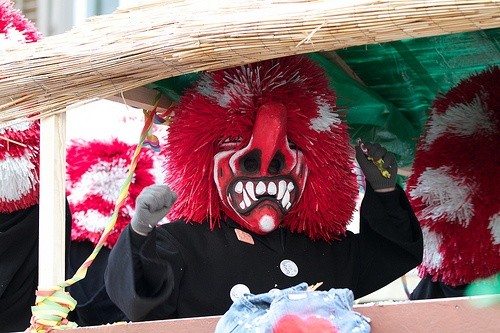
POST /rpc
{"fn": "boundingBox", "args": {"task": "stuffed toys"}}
[104,55,424,320]
[404,65,500,300]
[66,137,154,326]
[0,119,71,332]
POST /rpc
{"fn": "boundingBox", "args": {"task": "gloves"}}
[354,142,398,189]
[130,184,177,235]
